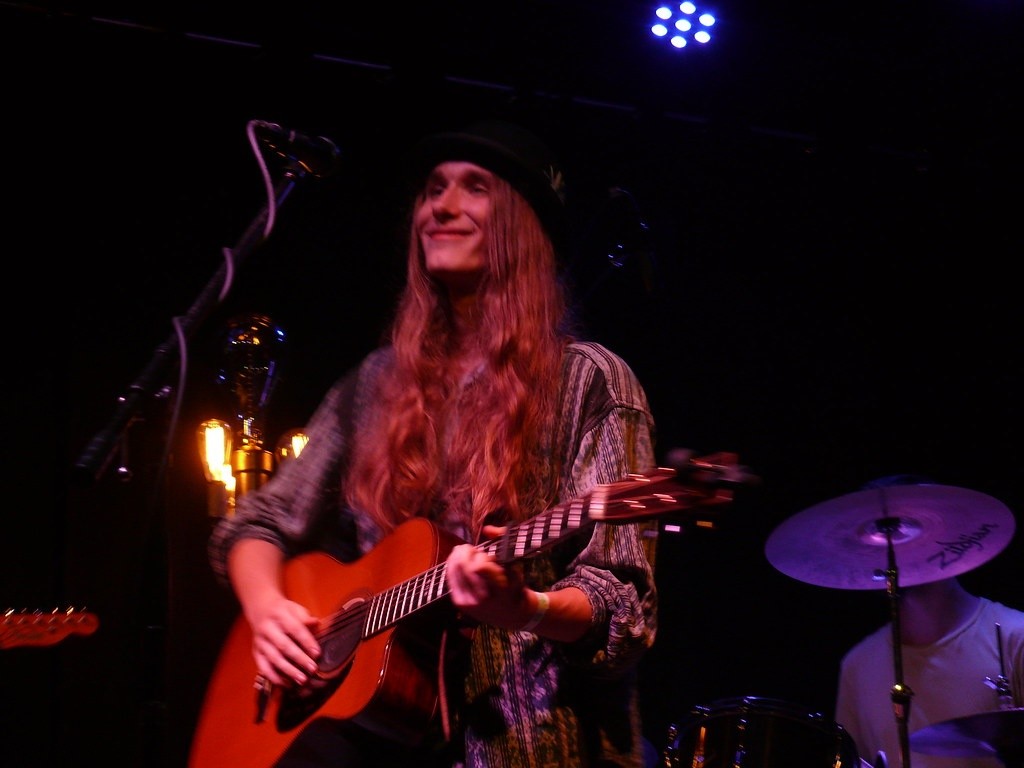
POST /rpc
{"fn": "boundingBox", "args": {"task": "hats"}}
[416,103,581,252]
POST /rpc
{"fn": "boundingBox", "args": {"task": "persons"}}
[835,573,1024,768]
[207,135,657,768]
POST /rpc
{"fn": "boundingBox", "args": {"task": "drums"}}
[662,694,859,768]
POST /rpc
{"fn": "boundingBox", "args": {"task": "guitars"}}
[0,604,98,649]
[191,443,746,768]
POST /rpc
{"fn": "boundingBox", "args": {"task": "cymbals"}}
[907,708,1024,756]
[765,482,1014,589]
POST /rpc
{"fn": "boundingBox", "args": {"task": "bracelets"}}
[519,589,550,632]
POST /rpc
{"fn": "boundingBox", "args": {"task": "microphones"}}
[257,121,343,163]
[622,193,659,288]
